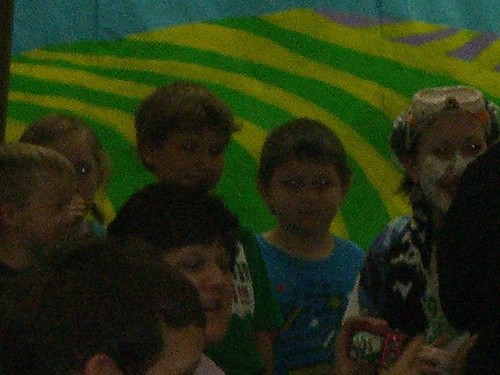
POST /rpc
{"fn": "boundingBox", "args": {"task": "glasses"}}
[403,85,493,158]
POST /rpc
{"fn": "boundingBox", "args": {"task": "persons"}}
[1,142,76,374]
[19,113,105,264]
[249,84,499,375]
[134,81,285,375]
[12,241,206,374]
[105,182,246,375]
